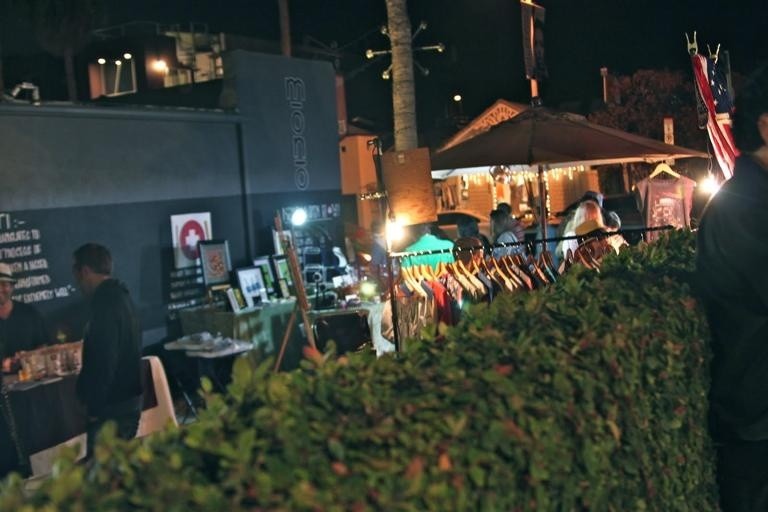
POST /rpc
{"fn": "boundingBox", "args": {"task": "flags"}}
[692,55,741,182]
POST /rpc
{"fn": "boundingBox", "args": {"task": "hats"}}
[576,219,605,236]
[0,262,17,283]
[584,190,604,208]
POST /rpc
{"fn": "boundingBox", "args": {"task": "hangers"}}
[388,230,623,297]
[631,159,697,194]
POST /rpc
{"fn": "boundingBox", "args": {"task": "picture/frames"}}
[197,238,235,290]
[249,256,278,296]
[235,266,267,308]
[275,275,292,299]
[226,287,245,313]
[271,256,292,295]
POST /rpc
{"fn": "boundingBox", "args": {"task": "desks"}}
[0,353,175,477]
[173,292,336,372]
[165,333,258,426]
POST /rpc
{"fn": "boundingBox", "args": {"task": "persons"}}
[72,244,143,460]
[452,236,482,270]
[370,219,401,292]
[0,262,50,360]
[491,190,629,265]
[700,67,767,511]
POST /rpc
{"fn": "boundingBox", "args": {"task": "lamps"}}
[284,204,308,253]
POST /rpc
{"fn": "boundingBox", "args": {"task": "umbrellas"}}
[430,98,710,252]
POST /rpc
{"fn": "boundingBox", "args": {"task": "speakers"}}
[338,133,382,196]
[381,147,439,227]
